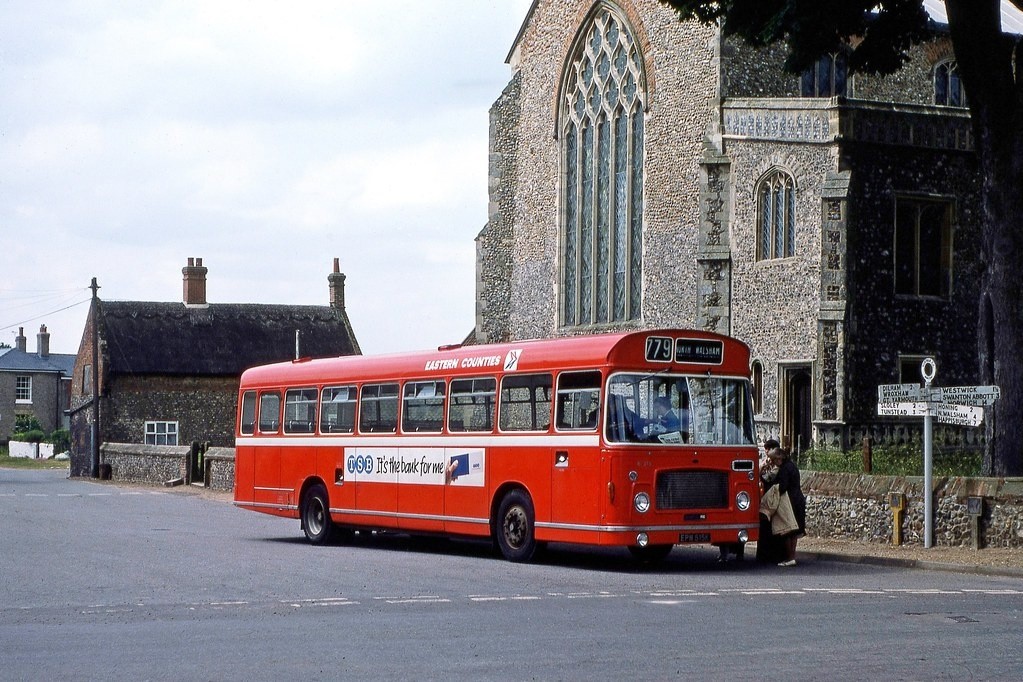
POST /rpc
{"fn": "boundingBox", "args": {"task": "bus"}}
[232,328,762,566]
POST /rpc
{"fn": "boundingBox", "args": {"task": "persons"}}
[758,439,808,566]
[653,397,681,443]
[445,458,459,485]
[588,394,653,443]
[711,541,746,562]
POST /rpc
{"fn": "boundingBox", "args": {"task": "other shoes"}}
[778,559,796,566]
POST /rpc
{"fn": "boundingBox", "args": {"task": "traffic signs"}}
[876,382,1000,426]
